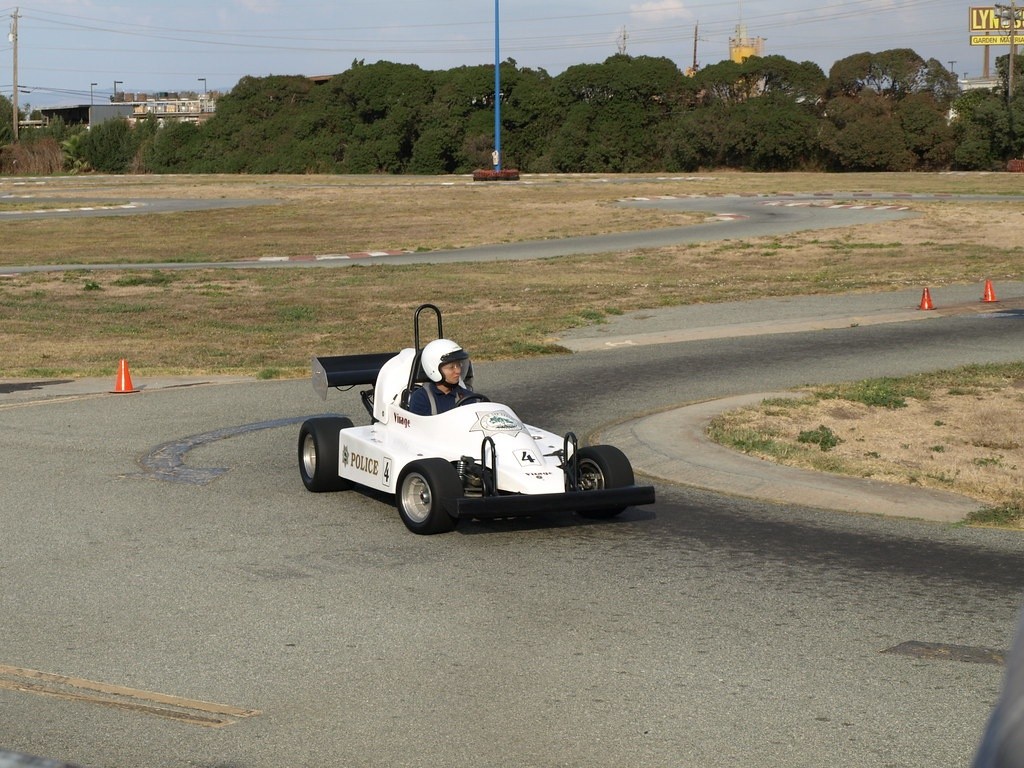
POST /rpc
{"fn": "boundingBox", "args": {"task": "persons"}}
[408,339,477,415]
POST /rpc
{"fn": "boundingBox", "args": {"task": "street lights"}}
[114,81,123,102]
[948,61,956,74]
[91,83,96,106]
[198,79,206,94]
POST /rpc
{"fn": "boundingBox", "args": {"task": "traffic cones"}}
[916,288,937,310]
[109,359,140,393]
[981,278,1000,302]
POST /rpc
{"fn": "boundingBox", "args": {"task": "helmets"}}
[421,339,470,384]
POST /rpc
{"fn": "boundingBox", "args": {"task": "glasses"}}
[442,363,462,371]
